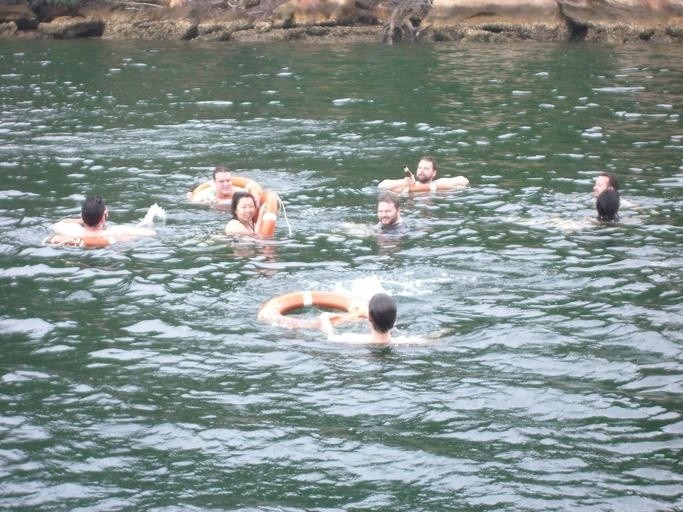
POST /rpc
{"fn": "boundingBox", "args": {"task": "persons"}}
[319,293,426,344]
[593,173,620,222]
[225,191,267,235]
[213,166,232,200]
[51,197,159,238]
[378,157,469,189]
[373,193,411,233]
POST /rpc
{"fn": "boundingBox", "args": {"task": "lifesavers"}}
[260,190,281,237]
[190,179,267,210]
[44,235,140,247]
[390,181,460,193]
[258,291,362,331]
